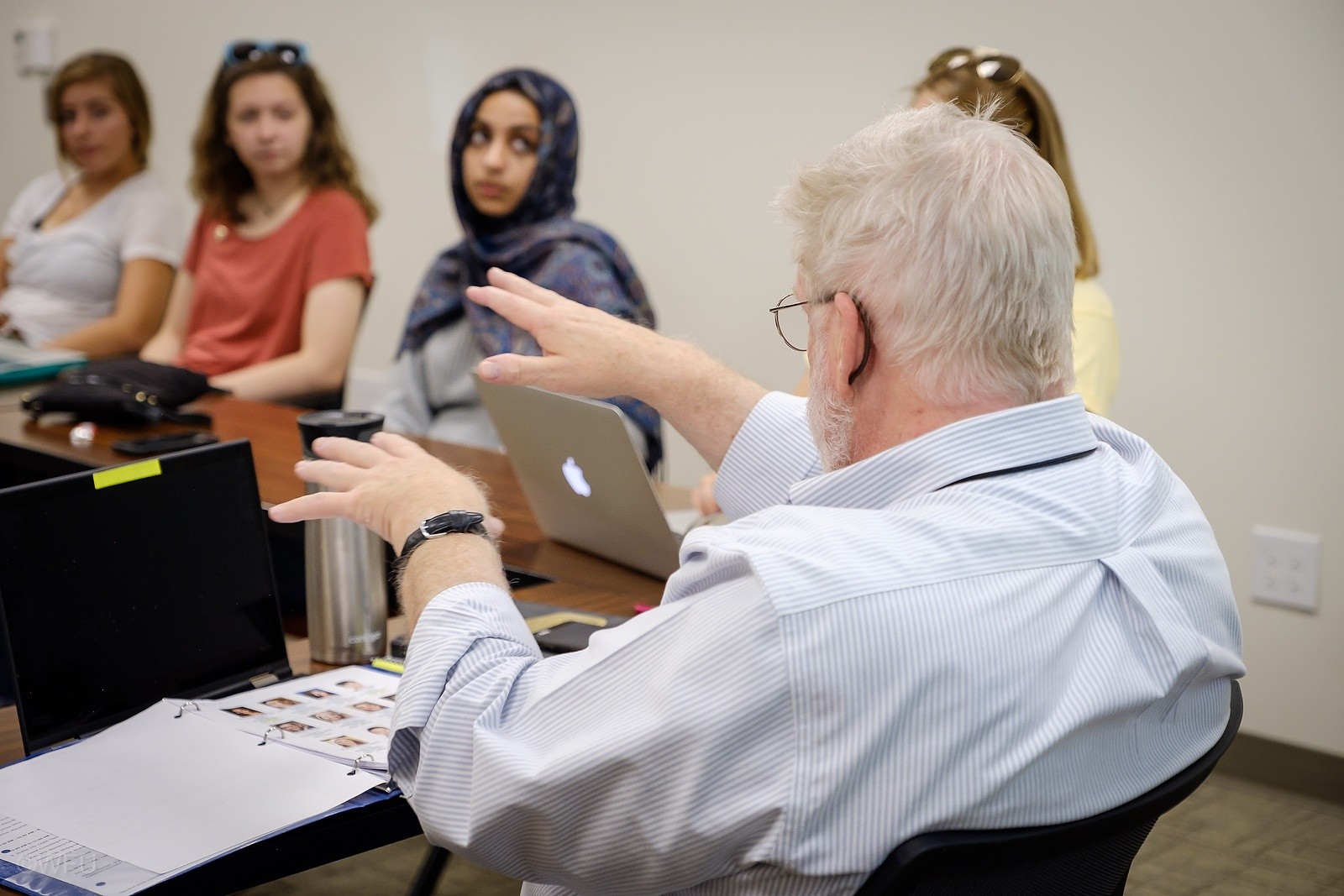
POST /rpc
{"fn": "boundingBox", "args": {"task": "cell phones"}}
[113,429,216,457]
[532,620,611,652]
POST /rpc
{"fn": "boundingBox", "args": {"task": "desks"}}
[0,371,699,896]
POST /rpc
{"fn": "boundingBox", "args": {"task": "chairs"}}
[855,678,1245,896]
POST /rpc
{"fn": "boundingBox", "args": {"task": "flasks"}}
[297,409,388,664]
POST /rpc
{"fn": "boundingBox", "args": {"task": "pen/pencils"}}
[370,655,406,675]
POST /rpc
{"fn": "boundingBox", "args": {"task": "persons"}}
[1,51,182,360]
[381,68,666,477]
[693,47,1120,517]
[270,103,1247,896]
[136,34,375,417]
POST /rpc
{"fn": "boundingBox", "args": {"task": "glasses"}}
[769,294,870,386]
[225,38,309,70]
[928,47,1025,87]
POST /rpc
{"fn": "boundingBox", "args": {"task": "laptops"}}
[470,367,687,580]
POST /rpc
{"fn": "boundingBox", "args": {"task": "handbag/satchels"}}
[21,357,208,432]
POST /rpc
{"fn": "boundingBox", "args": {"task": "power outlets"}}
[1248,523,1321,613]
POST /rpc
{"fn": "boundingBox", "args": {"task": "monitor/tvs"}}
[0,435,295,757]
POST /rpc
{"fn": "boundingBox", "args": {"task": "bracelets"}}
[397,510,497,577]
